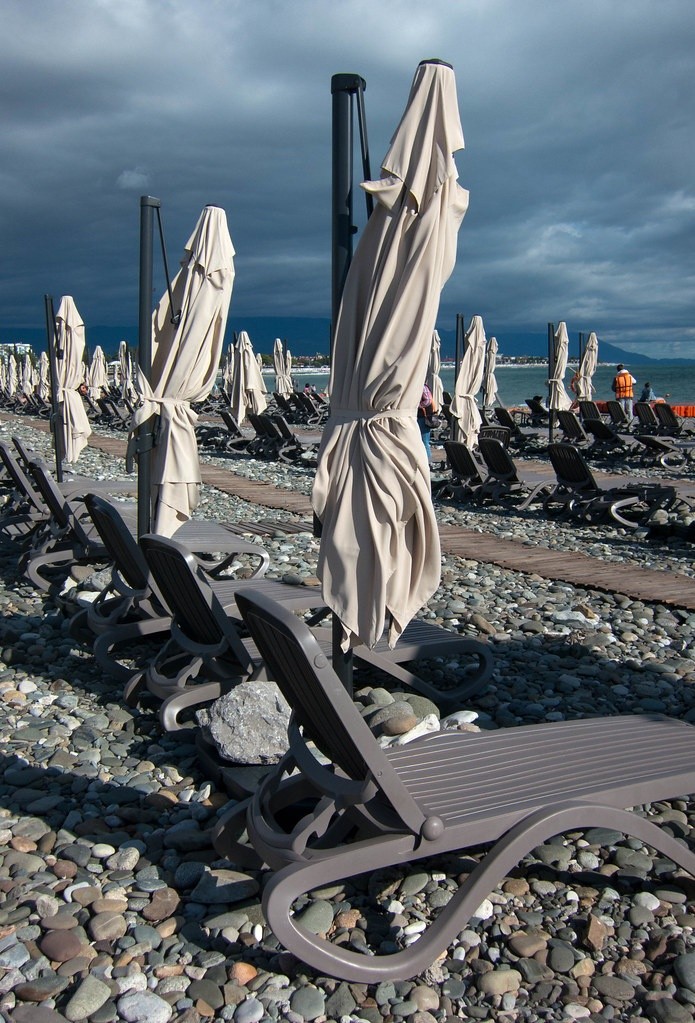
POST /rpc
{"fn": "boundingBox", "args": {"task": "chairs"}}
[83,386,142,431]
[429,391,695,539]
[0,384,53,419]
[0,434,695,983]
[191,388,330,467]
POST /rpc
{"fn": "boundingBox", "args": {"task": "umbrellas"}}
[223,331,267,428]
[273,338,293,400]
[113,341,137,409]
[311,58,469,703]
[548,321,573,442]
[0,351,50,401]
[482,336,498,413]
[453,315,487,450]
[45,293,92,484]
[136,194,235,552]
[82,345,109,387]
[579,331,598,423]
[427,329,445,415]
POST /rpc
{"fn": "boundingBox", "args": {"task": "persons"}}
[417,385,433,458]
[612,365,636,420]
[311,385,316,392]
[304,384,310,393]
[79,385,106,397]
[533,396,549,412]
[641,382,655,401]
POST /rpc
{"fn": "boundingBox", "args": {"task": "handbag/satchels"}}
[424,414,440,428]
